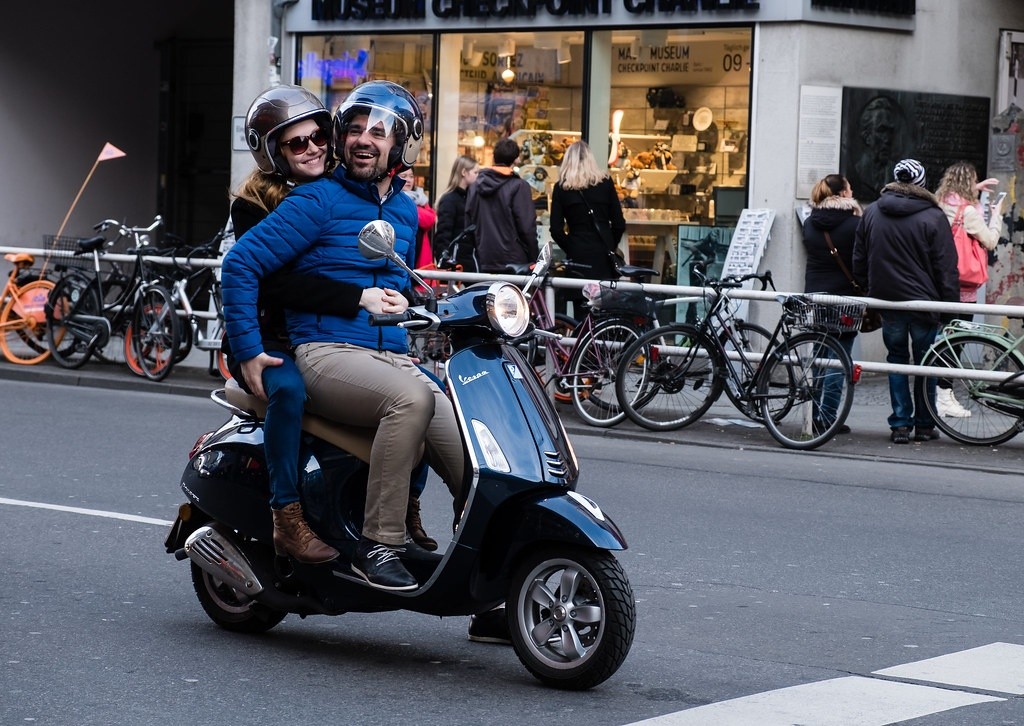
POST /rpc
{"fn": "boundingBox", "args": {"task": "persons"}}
[802,175,862,434]
[935,163,1003,417]
[685,231,718,323]
[549,140,626,337]
[852,159,960,444]
[220,79,511,645]
[397,168,438,292]
[464,140,539,273]
[433,155,479,271]
[230,85,439,562]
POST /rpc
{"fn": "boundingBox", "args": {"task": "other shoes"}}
[822,421,851,435]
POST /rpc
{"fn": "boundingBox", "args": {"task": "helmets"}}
[245,86,332,174]
[332,80,424,173]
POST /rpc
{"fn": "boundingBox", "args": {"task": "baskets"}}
[43,234,95,271]
[599,279,666,320]
[789,291,867,336]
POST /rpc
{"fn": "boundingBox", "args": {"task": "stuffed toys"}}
[608,141,677,208]
[514,132,574,200]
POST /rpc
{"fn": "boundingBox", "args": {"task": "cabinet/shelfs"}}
[507,130,699,236]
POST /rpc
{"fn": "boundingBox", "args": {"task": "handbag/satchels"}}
[860,309,882,333]
[951,202,989,290]
[608,247,625,281]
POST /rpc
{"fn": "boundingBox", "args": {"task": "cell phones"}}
[991,192,1007,207]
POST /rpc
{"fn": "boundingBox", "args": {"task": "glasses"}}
[282,128,327,155]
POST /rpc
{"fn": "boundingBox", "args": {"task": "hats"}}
[894,160,925,189]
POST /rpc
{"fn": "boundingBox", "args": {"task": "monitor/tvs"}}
[713,186,746,226]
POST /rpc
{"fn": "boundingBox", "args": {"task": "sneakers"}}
[352,537,419,590]
[469,608,510,643]
[916,427,939,441]
[891,427,910,443]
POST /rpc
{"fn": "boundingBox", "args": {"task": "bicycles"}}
[0,253,69,364]
[6,215,234,382]
[406,225,868,451]
[919,319,1024,446]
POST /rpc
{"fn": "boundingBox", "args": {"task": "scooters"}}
[164,220,636,690]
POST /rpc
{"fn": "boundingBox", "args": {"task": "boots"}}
[405,497,439,552]
[270,503,340,564]
[936,387,971,417]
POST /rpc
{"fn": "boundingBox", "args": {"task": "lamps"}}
[497,39,515,57]
[463,39,473,60]
[501,60,515,83]
[630,40,640,56]
[557,39,571,64]
